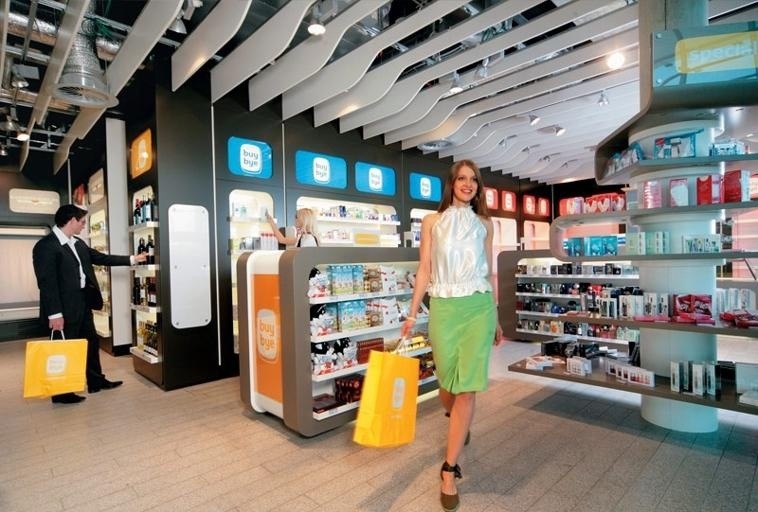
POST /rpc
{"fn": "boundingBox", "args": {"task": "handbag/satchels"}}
[23,339,88,398]
[351,348,421,447]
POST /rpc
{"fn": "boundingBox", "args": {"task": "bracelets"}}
[407,316,416,321]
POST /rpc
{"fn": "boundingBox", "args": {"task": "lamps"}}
[306,4,326,36]
[11,67,31,143]
[169,9,188,35]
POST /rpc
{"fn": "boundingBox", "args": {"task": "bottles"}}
[130,194,159,354]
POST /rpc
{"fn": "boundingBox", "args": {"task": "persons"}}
[400,160,503,512]
[32,204,149,404]
[267,208,319,247]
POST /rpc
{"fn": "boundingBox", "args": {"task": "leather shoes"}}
[51,393,86,403]
[89,377,122,393]
[440,462,460,511]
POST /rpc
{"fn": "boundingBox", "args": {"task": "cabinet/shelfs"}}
[230,189,403,252]
[308,261,442,418]
[496,86,757,434]
[125,187,160,367]
